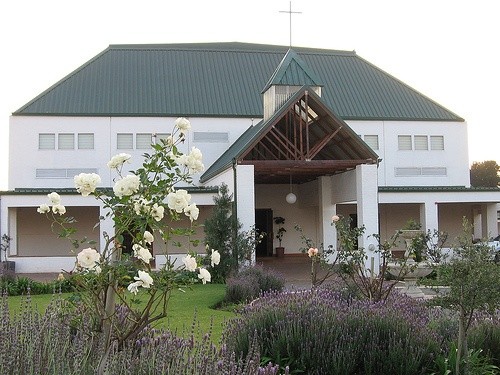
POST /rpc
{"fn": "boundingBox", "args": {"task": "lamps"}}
[285,173,296,204]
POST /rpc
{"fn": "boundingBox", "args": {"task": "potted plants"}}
[273,217,286,257]
